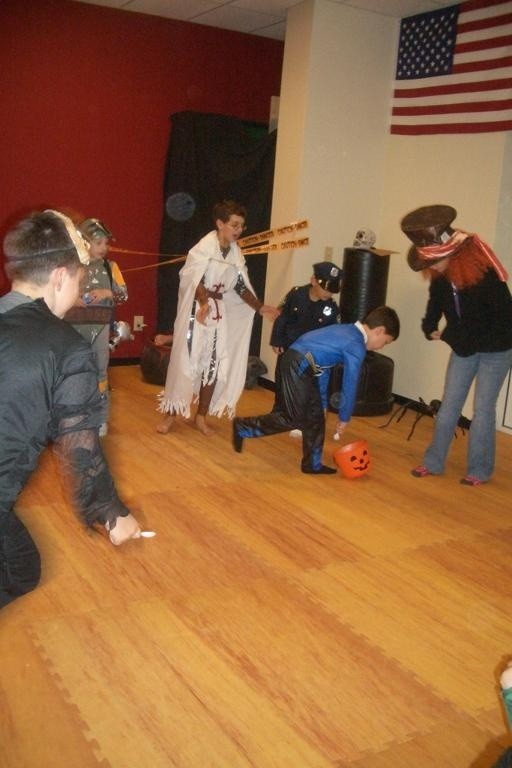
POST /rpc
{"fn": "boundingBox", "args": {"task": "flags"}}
[390,0,511,135]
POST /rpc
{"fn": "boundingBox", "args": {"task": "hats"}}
[314,262,343,293]
[402,205,472,271]
[83,214,111,242]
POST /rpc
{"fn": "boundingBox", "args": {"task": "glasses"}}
[226,224,247,231]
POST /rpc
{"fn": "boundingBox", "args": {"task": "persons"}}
[1,208,146,611]
[268,261,345,439]
[155,200,281,437]
[230,305,401,475]
[63,216,130,438]
[400,204,511,487]
